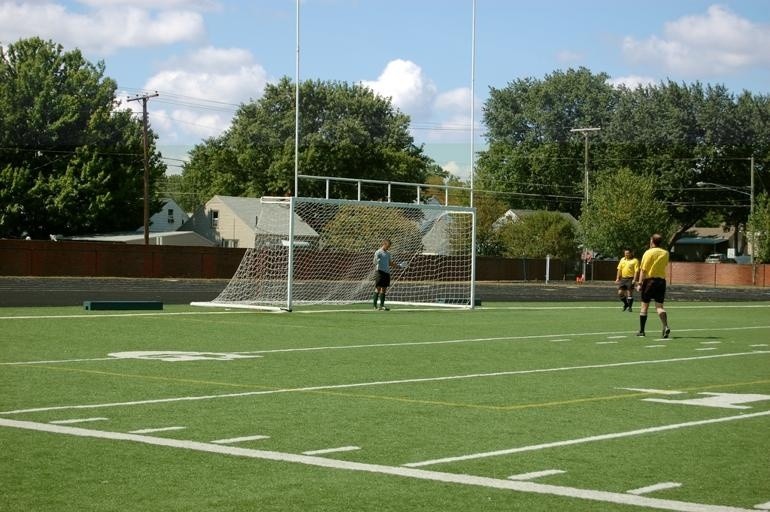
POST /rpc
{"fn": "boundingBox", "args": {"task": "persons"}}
[636,234,670,338]
[615,248,640,312]
[372,240,407,311]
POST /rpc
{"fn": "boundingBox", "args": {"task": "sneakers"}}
[636,332,645,337]
[622,303,633,312]
[662,326,671,338]
[373,306,390,311]
